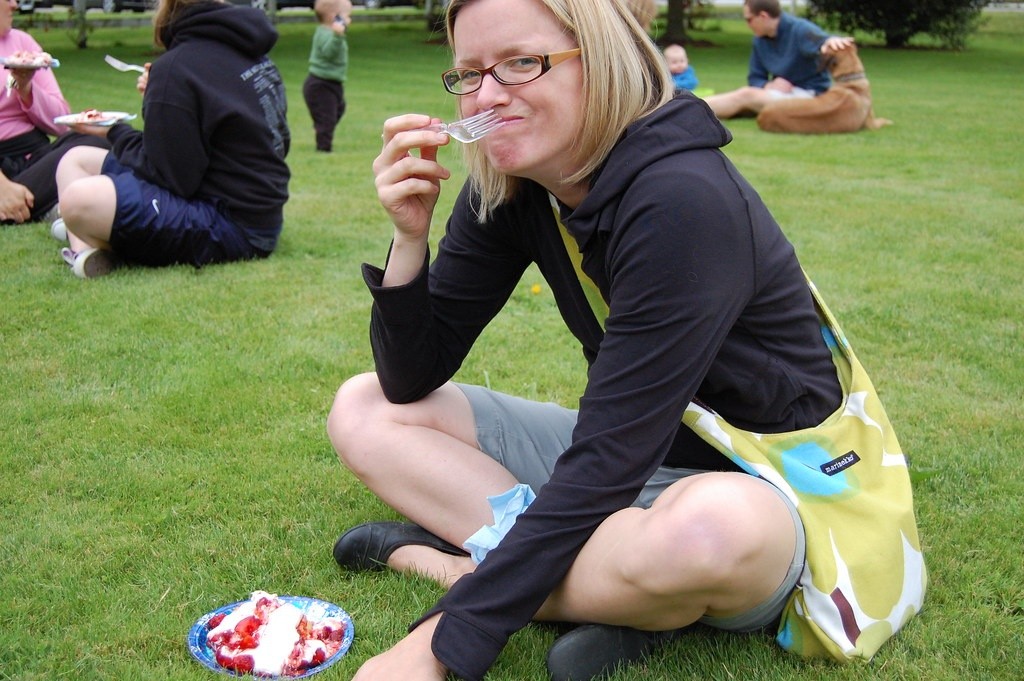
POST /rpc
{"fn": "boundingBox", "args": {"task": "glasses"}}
[442,48,582,96]
[745,15,756,23]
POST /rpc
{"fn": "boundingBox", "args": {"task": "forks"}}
[408,109,506,143]
[105,55,145,74]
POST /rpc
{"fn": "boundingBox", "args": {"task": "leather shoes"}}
[546,622,654,681]
[332,521,471,575]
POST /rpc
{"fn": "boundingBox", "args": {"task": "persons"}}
[699,0,855,120]
[51,0,291,279]
[327,0,844,681]
[303,0,352,152]
[0,0,111,225]
[663,45,698,90]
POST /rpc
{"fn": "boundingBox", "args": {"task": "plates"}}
[2,60,60,68]
[188,596,354,681]
[57,112,128,123]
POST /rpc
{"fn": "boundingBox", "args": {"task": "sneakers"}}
[51,217,68,241]
[61,247,116,280]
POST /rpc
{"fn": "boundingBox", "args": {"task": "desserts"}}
[4,50,52,66]
[77,109,103,122]
[207,590,344,677]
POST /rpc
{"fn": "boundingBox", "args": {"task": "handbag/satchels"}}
[674,325,928,667]
